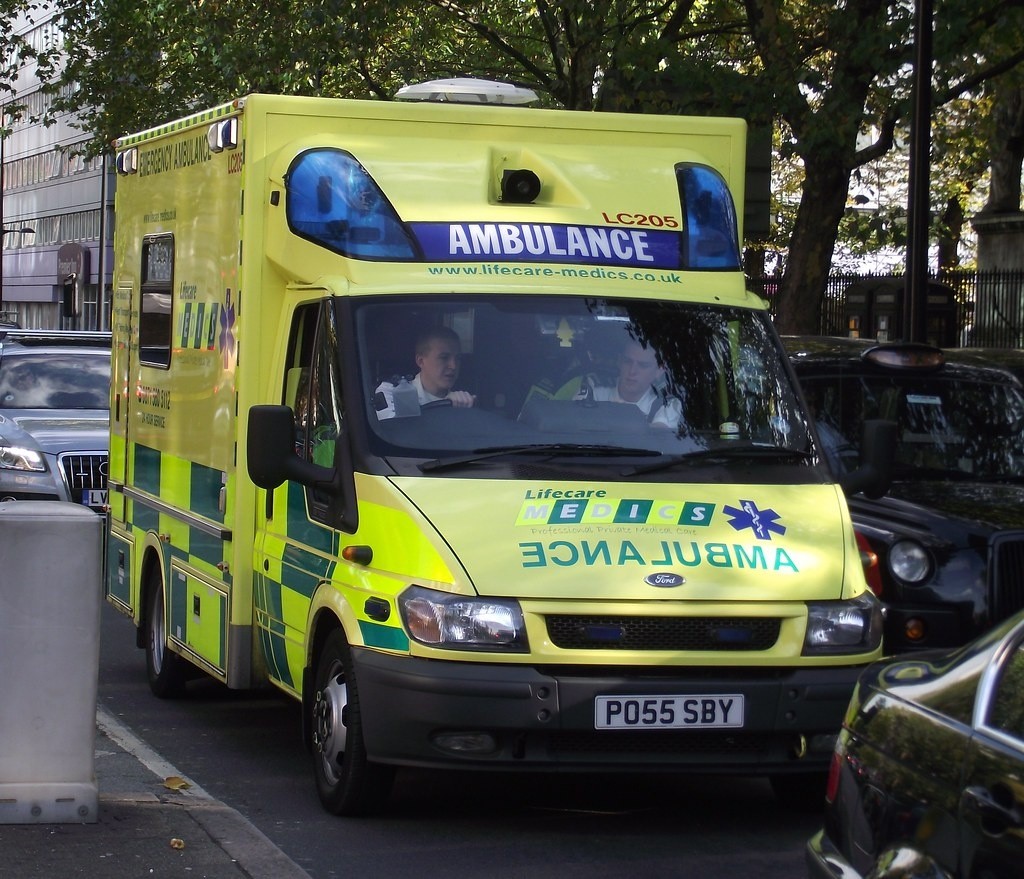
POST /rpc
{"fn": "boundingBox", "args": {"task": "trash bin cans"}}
[0,500,103,823]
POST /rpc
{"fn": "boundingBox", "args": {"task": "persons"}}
[577,334,681,431]
[375,327,476,422]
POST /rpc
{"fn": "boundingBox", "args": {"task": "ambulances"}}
[104,73,891,822]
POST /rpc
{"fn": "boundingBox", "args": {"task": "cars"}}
[1,308,108,518]
[773,333,1024,655]
[802,608,1024,878]
